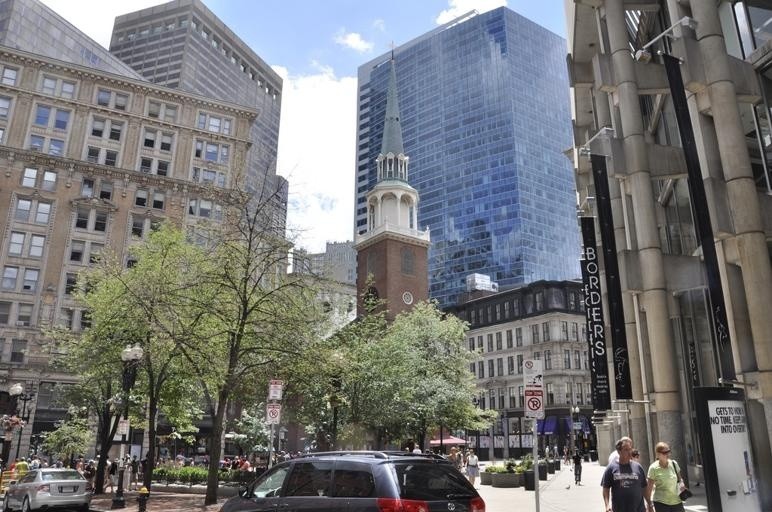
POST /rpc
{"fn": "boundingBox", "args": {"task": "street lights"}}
[111,342,144,510]
[8,382,37,466]
[570,407,580,452]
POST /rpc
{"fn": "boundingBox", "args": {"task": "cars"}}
[3,468,94,512]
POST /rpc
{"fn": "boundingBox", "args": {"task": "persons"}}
[600,435,693,512]
[545,445,582,487]
[404,444,480,489]
[1,450,302,493]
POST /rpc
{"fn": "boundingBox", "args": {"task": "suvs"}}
[218,449,486,512]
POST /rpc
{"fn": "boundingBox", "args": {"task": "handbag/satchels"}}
[679,489,693,501]
